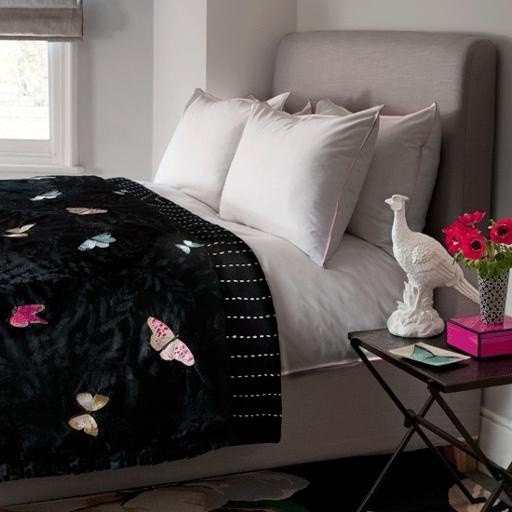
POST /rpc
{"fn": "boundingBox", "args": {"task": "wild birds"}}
[382,193,482,320]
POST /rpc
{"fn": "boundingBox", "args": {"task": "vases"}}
[478,271,509,323]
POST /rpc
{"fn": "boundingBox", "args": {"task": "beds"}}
[0,32,498,512]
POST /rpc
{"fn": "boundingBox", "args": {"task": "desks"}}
[345,328,512,510]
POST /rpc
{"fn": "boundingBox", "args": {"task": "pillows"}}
[149,87,291,212]
[217,101,387,271]
[244,92,313,116]
[314,97,438,261]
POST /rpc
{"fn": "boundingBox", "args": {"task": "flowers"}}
[445,209,512,274]
